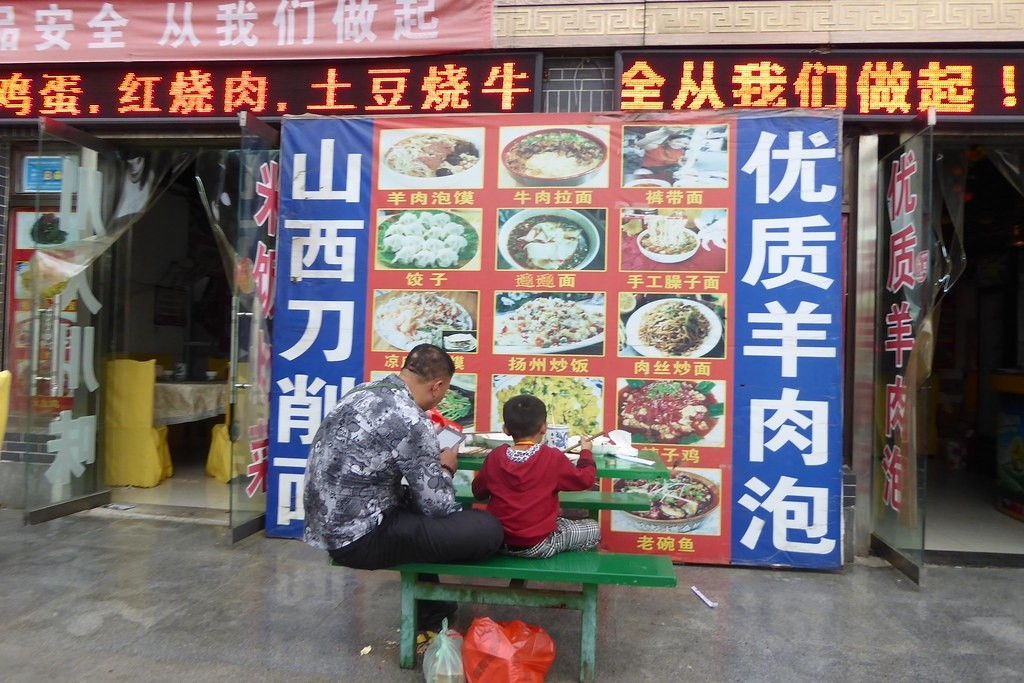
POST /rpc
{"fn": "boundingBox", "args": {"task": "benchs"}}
[332,483,678,683]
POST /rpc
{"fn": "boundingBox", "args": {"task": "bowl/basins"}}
[482,434,515,449]
[614,470,719,533]
[501,128,608,186]
[380,131,481,185]
[498,208,600,270]
[619,294,636,315]
[636,228,701,264]
[164,370,174,375]
[205,371,218,380]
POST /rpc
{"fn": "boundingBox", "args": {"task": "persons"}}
[470,394,603,559]
[303,344,505,631]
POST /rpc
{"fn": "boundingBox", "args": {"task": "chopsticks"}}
[465,446,485,454]
[562,431,606,453]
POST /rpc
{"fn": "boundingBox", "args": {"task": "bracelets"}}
[440,463,455,478]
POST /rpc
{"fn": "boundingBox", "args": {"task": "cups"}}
[545,424,568,452]
[175,363,186,380]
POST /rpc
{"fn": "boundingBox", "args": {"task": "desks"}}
[440,442,670,479]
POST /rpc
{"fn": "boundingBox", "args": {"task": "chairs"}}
[207,357,270,485]
[91,352,174,489]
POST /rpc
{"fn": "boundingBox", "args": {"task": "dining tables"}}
[152,383,227,427]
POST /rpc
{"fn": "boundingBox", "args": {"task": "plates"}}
[625,298,722,359]
[376,208,480,269]
[457,447,492,458]
[16,261,76,299]
[492,304,604,354]
[492,375,603,436]
[15,316,75,351]
[624,166,729,188]
[564,453,580,463]
[16,360,72,397]
[374,296,473,351]
